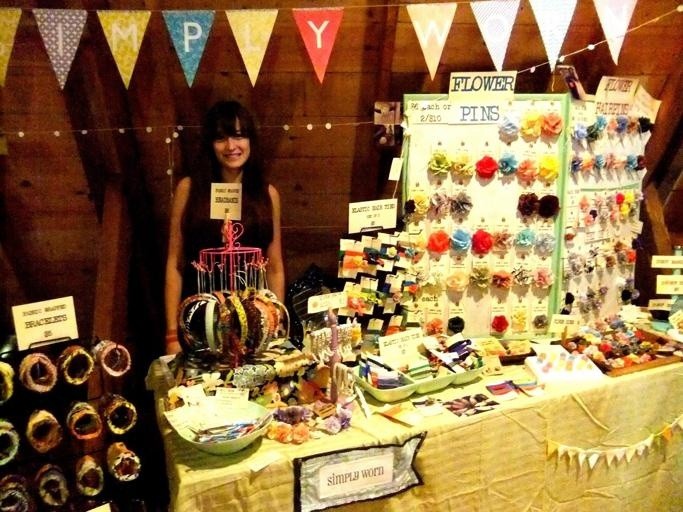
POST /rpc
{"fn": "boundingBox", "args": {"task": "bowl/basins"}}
[161,395,271,457]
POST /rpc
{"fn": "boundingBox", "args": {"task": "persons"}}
[163,99,285,355]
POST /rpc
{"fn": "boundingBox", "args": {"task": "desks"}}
[145,329,683,512]
